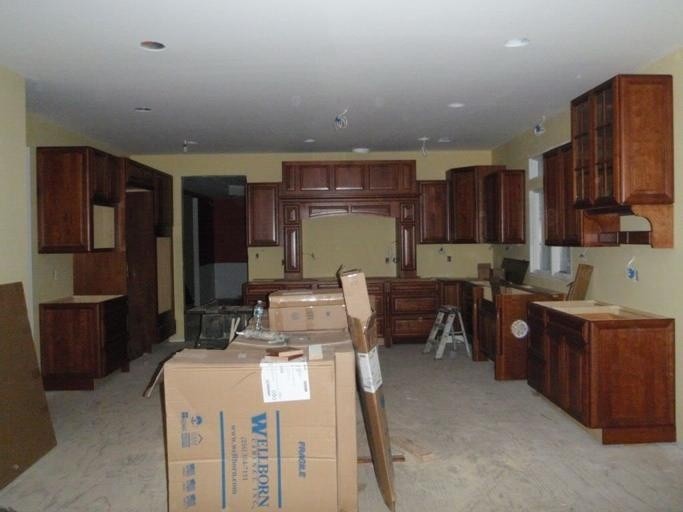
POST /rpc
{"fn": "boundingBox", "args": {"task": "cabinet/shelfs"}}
[154,170,174,237]
[448,168,526,243]
[123,162,152,355]
[390,280,443,337]
[243,283,391,344]
[439,280,463,335]
[462,281,482,361]
[282,161,416,195]
[528,301,675,445]
[38,296,123,389]
[418,182,448,245]
[246,183,281,247]
[542,142,618,248]
[569,75,673,207]
[37,146,119,253]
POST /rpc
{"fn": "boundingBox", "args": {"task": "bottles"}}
[250,299,264,332]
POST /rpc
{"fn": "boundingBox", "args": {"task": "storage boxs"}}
[163,264,397,512]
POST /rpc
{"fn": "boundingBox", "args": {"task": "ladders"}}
[424,304,471,360]
